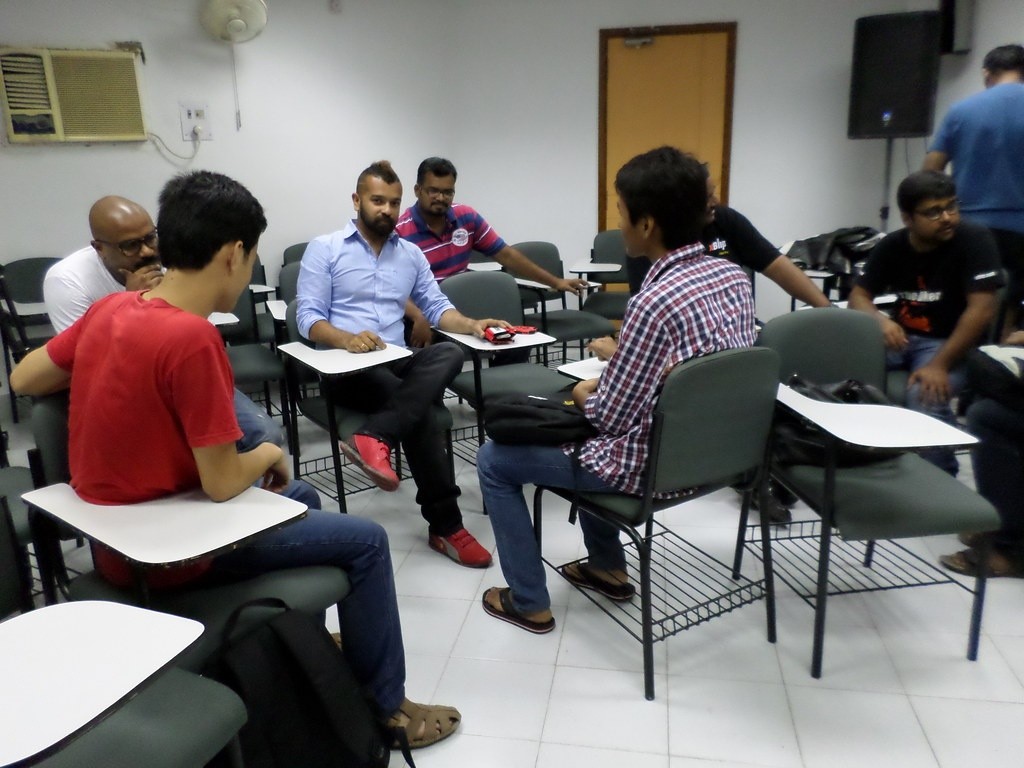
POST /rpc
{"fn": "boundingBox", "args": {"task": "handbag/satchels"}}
[481,391,605,448]
[770,373,913,469]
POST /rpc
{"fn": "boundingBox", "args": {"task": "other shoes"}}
[745,474,800,525]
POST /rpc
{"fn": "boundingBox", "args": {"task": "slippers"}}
[563,562,636,599]
[482,588,556,633]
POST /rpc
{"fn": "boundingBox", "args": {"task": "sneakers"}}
[340,431,398,491]
[428,525,492,568]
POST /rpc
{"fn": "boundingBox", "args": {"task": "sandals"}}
[938,541,1024,577]
[958,533,985,546]
[391,696,462,749]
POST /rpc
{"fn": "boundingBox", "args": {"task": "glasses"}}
[96,226,157,258]
[420,184,456,200]
[912,199,963,221]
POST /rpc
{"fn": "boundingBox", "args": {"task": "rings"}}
[360,343,365,348]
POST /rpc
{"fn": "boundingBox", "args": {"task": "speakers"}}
[846,10,941,139]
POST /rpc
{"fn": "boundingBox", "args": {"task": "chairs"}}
[0,226,1024,767]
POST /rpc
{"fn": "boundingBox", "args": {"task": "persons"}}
[396,157,592,351]
[850,170,1005,475]
[474,146,755,635]
[625,163,840,527]
[10,170,461,749]
[941,331,1024,577]
[923,43,1024,346]
[296,160,515,566]
[43,195,284,486]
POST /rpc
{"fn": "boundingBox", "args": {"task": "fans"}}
[199,0,269,128]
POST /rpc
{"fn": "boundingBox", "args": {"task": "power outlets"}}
[180,103,213,141]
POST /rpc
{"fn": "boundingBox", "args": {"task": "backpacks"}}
[199,597,389,768]
